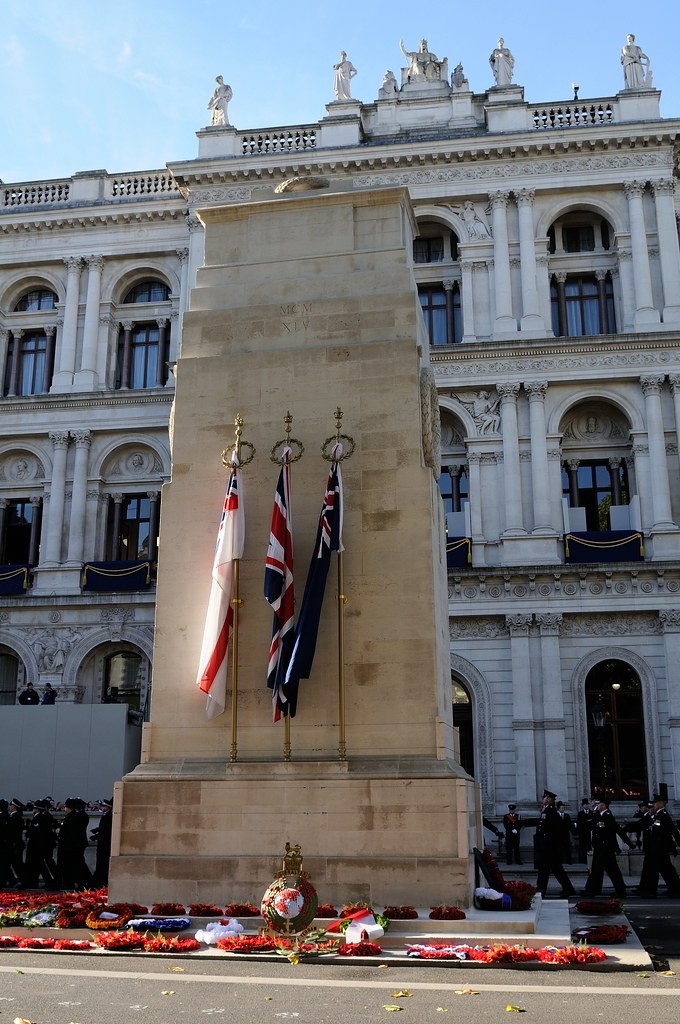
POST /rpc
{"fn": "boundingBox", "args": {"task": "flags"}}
[274,444,345,718]
[197,452,245,722]
[261,446,296,721]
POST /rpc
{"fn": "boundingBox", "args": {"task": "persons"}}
[445,200,494,241]
[18,682,39,705]
[332,50,357,100]
[489,37,515,85]
[41,683,57,705]
[482,816,499,836]
[207,75,233,125]
[0,796,113,892]
[515,790,680,901]
[452,391,502,435]
[503,804,523,865]
[400,38,448,81]
[621,33,650,89]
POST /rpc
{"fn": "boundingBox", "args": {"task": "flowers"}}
[571,924,632,945]
[427,901,467,920]
[187,901,223,917]
[340,898,392,934]
[569,898,631,918]
[404,940,608,966]
[382,902,418,920]
[88,925,201,953]
[150,900,186,916]
[314,903,337,919]
[225,899,261,918]
[215,926,384,965]
[0,885,148,931]
[0,935,92,951]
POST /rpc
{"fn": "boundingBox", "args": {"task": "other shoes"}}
[663,890,680,897]
[533,891,546,896]
[608,891,626,896]
[579,889,601,895]
[507,862,512,865]
[631,889,643,894]
[0,880,49,890]
[557,891,576,898]
[516,861,524,865]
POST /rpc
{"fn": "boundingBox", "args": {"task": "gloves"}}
[513,830,517,833]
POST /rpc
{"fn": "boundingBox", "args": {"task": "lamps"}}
[591,696,609,730]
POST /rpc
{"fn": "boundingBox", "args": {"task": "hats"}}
[33,800,47,810]
[100,799,113,809]
[542,789,557,801]
[652,793,668,803]
[637,799,656,808]
[589,792,612,806]
[65,798,76,809]
[75,797,87,809]
[11,797,24,809]
[556,800,566,809]
[581,798,591,806]
[42,797,54,811]
[508,803,517,809]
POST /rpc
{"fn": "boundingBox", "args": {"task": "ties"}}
[585,809,587,814]
[560,813,563,818]
[511,813,513,816]
